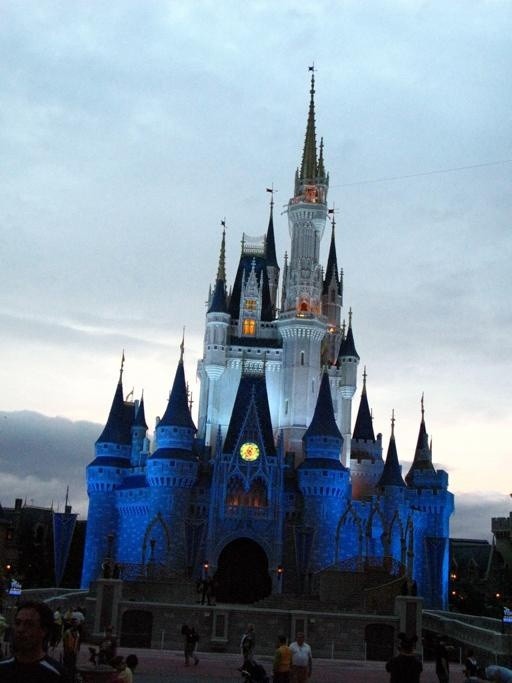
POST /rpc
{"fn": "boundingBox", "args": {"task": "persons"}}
[208,576,217,605]
[197,577,209,604]
[271,635,292,683]
[239,623,256,671]
[449,587,512,619]
[402,575,417,596]
[0,560,139,683]
[290,632,312,683]
[385,639,422,683]
[436,634,449,683]
[181,623,199,666]
[461,647,512,683]
